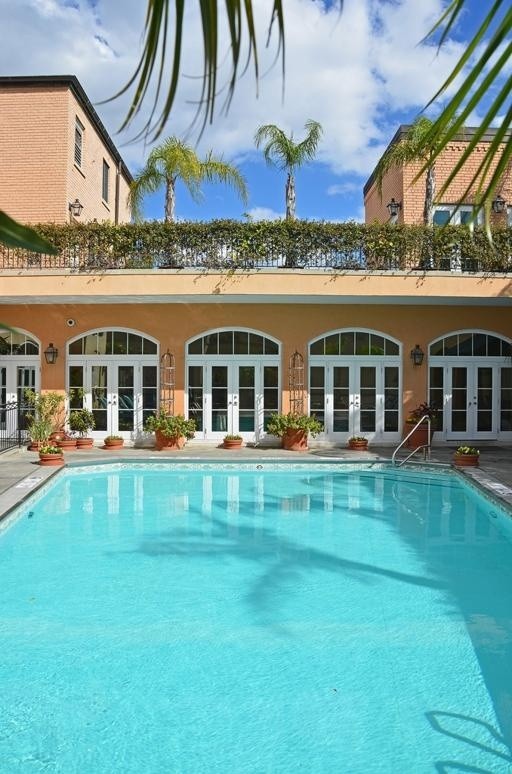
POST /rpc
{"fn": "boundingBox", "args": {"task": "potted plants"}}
[104,435,123,450]
[405,401,440,450]
[454,446,480,466]
[23,389,96,466]
[143,406,198,450]
[224,434,242,449]
[349,435,368,451]
[266,411,325,451]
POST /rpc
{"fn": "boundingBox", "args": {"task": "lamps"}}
[493,195,506,212]
[43,343,58,365]
[410,344,424,366]
[386,198,402,216]
[71,198,84,216]
